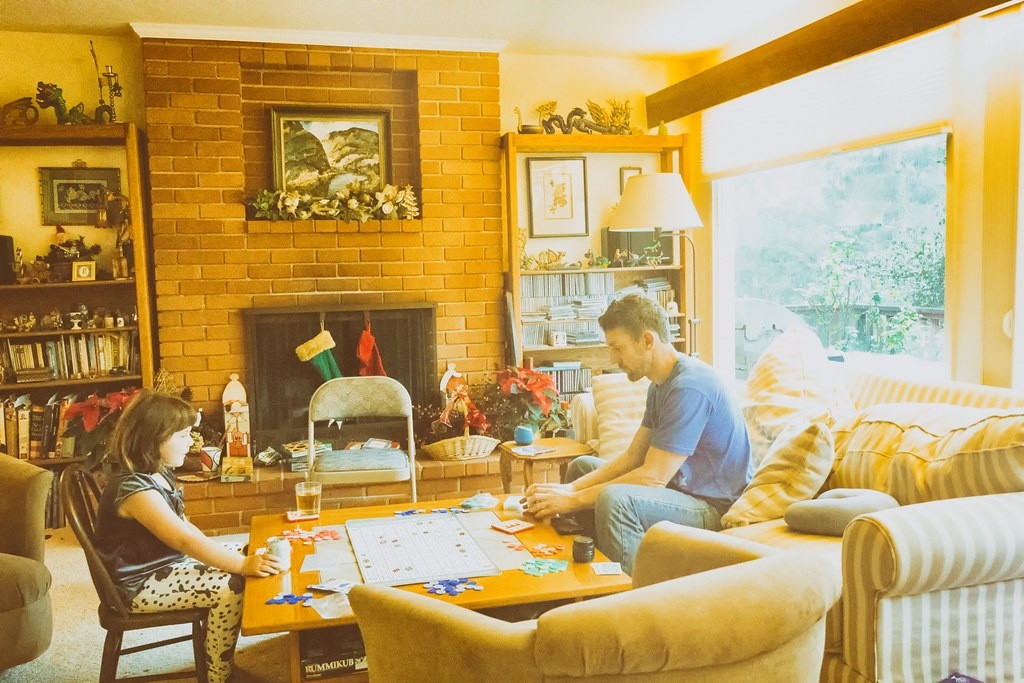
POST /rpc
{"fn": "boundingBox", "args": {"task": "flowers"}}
[239,183,408,221]
[489,367,560,430]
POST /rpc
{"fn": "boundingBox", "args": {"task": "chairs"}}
[59,465,210,683]
[307,375,417,504]
[0,453,53,671]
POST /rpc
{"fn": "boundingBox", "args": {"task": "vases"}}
[514,424,535,444]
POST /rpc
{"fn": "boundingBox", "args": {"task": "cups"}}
[268,536,291,572]
[201,447,222,472]
[515,426,533,445]
[295,481,321,514]
[573,538,594,562]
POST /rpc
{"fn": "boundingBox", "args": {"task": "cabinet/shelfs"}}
[0,122,153,465]
[501,132,694,368]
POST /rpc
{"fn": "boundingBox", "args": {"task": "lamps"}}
[609,172,704,358]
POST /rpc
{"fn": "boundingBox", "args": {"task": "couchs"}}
[571,324,1023,682]
[348,520,844,683]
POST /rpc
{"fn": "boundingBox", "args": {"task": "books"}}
[0,387,140,461]
[524,355,592,402]
[44,469,71,528]
[521,273,681,347]
[344,438,400,449]
[283,439,332,472]
[0,331,137,379]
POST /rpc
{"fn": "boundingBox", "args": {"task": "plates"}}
[177,473,220,482]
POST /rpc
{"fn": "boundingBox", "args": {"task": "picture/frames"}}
[72,261,95,282]
[526,156,588,239]
[269,105,395,197]
[38,166,122,225]
[620,167,642,195]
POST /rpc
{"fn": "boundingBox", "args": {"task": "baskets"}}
[420,399,501,460]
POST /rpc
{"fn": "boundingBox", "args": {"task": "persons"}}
[93,392,284,683]
[519,293,751,578]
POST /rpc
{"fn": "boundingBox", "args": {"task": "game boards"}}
[344,512,502,587]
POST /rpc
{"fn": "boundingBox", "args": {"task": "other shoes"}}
[225,662,269,683]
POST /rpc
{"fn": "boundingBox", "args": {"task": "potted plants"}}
[182,431,203,470]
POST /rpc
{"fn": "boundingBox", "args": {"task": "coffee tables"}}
[240,494,632,683]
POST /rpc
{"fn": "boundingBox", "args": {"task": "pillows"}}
[720,411,835,527]
[592,372,651,460]
[739,326,833,484]
[784,488,901,536]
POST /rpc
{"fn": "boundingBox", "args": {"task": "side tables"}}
[498,437,591,494]
